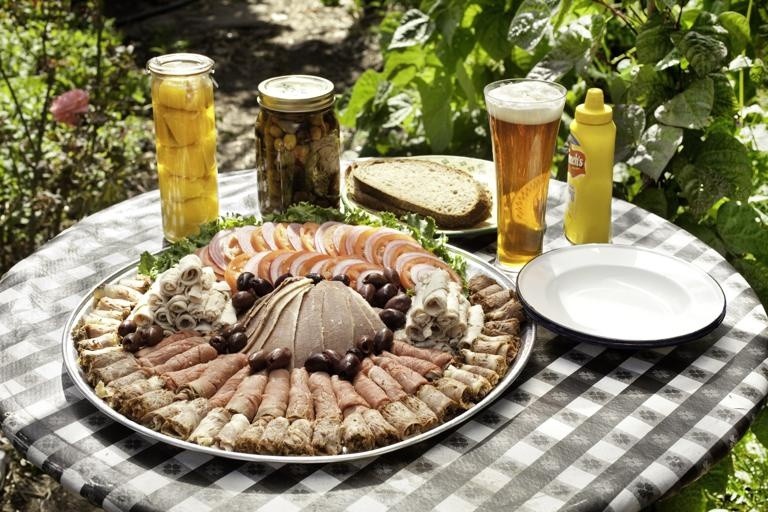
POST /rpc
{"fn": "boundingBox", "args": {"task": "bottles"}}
[564,86,621,246]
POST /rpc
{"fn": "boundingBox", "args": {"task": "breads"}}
[354,157,493,226]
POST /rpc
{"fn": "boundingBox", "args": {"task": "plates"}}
[514,241,729,347]
[57,234,538,465]
[340,151,498,236]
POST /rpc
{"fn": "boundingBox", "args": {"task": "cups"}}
[480,74,568,265]
